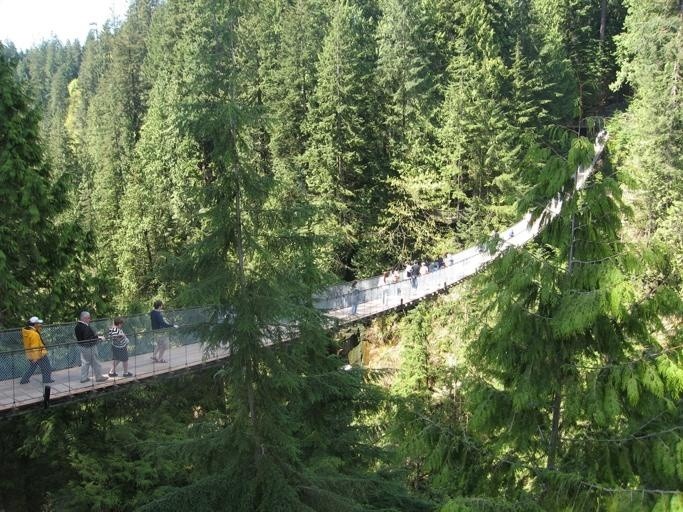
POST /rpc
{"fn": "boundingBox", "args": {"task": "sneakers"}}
[20,379,55,384]
[151,356,168,363]
[81,372,132,383]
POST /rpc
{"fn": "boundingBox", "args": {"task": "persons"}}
[74,311,108,382]
[495,231,500,238]
[108,319,132,377]
[349,280,360,315]
[393,271,401,295]
[510,230,514,237]
[150,301,177,364]
[379,272,392,306]
[404,253,454,293]
[19,316,55,384]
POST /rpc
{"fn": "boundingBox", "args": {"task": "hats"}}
[30,316,43,324]
